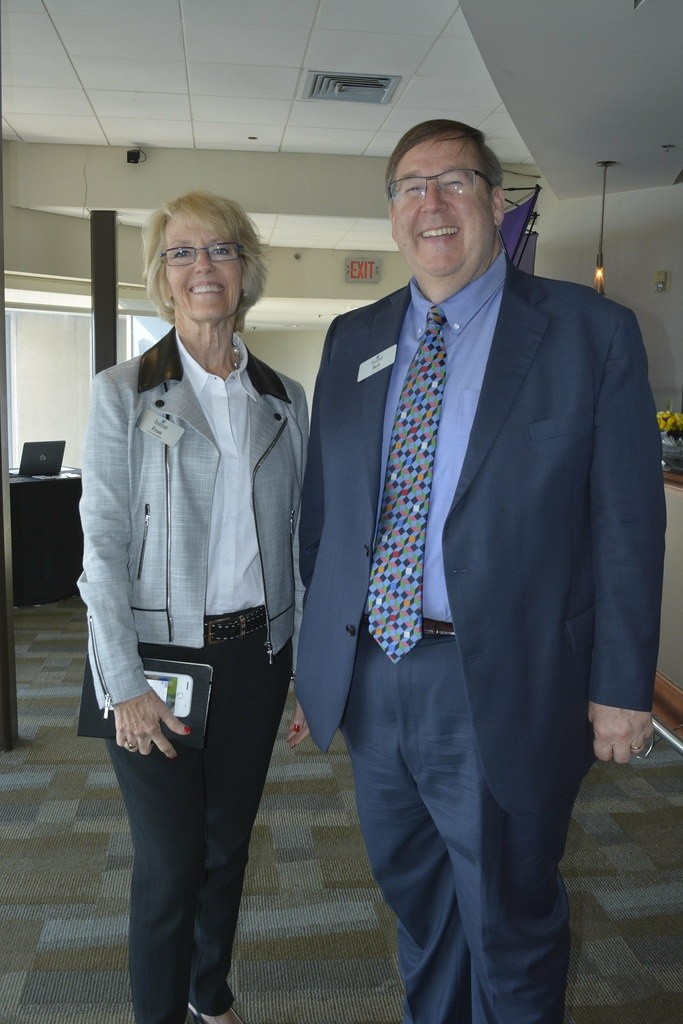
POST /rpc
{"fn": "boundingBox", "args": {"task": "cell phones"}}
[143,670,193,717]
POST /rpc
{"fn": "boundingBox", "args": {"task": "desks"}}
[9,466,83,608]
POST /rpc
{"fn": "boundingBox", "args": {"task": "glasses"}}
[388,169,493,206]
[161,242,240,266]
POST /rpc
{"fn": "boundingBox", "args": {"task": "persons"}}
[75,195,311,1024]
[294,121,666,1024]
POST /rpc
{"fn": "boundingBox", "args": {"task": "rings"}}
[630,743,646,751]
[123,741,137,750]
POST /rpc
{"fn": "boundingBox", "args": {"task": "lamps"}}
[594,160,617,296]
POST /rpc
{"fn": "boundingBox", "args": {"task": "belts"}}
[202,606,267,645]
[363,616,453,636]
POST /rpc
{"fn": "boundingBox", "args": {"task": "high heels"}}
[188,1003,243,1024]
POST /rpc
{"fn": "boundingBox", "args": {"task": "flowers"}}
[656,410,683,446]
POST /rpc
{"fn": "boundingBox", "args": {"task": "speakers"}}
[127,150,139,163]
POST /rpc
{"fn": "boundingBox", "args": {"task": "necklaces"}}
[232,341,241,371]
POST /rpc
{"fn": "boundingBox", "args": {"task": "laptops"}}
[9,440,66,476]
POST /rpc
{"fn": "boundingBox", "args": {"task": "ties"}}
[367,306,449,664]
